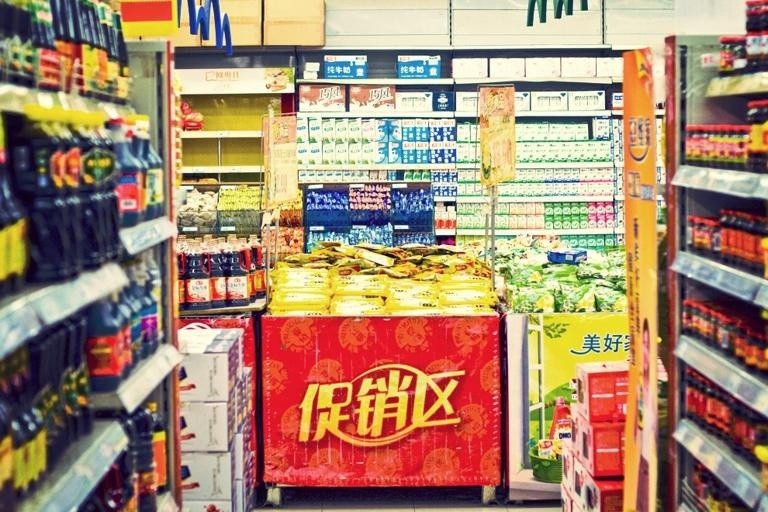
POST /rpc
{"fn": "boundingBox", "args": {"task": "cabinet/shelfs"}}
[175,44,666,256]
[1,1,180,511]
[663,2,767,511]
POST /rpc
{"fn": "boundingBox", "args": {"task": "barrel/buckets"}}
[174,231,272,309]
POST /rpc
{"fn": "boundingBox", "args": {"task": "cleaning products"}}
[548,395,572,441]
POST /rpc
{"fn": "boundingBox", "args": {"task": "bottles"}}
[176,234,267,309]
[1,0,173,510]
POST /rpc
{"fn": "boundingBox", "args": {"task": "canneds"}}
[680,0,768,512]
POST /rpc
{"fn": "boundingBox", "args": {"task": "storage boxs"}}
[560,358,648,510]
[176,322,258,511]
